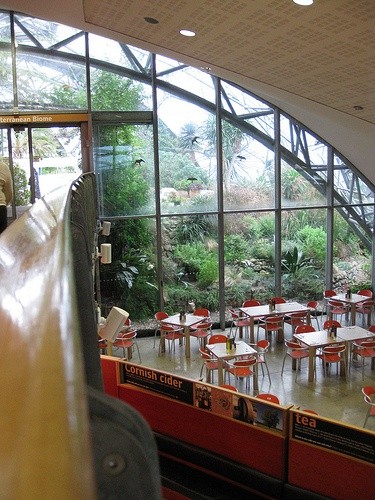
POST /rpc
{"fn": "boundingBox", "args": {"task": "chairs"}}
[258,316,285,346]
[157,324,185,360]
[314,343,350,385]
[226,308,250,340]
[285,311,309,332]
[241,299,265,337]
[206,333,228,343]
[322,318,341,330]
[324,288,373,326]
[97,335,109,355]
[114,327,142,364]
[361,384,375,429]
[199,347,230,385]
[306,301,321,331]
[194,307,211,318]
[279,337,310,382]
[153,311,177,350]
[189,321,214,352]
[272,297,286,303]
[123,318,133,329]
[350,325,375,381]
[222,356,259,394]
[244,339,272,384]
[293,323,315,333]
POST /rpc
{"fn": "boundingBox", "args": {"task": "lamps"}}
[95,243,112,264]
[98,221,111,236]
[95,302,129,345]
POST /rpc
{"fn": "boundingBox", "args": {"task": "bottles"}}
[327,322,338,340]
[269,299,275,312]
[345,287,352,300]
[226,334,237,352]
[179,307,186,321]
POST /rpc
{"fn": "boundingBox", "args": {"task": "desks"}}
[238,301,311,347]
[293,326,375,382]
[204,340,260,394]
[159,313,209,358]
[330,292,370,326]
